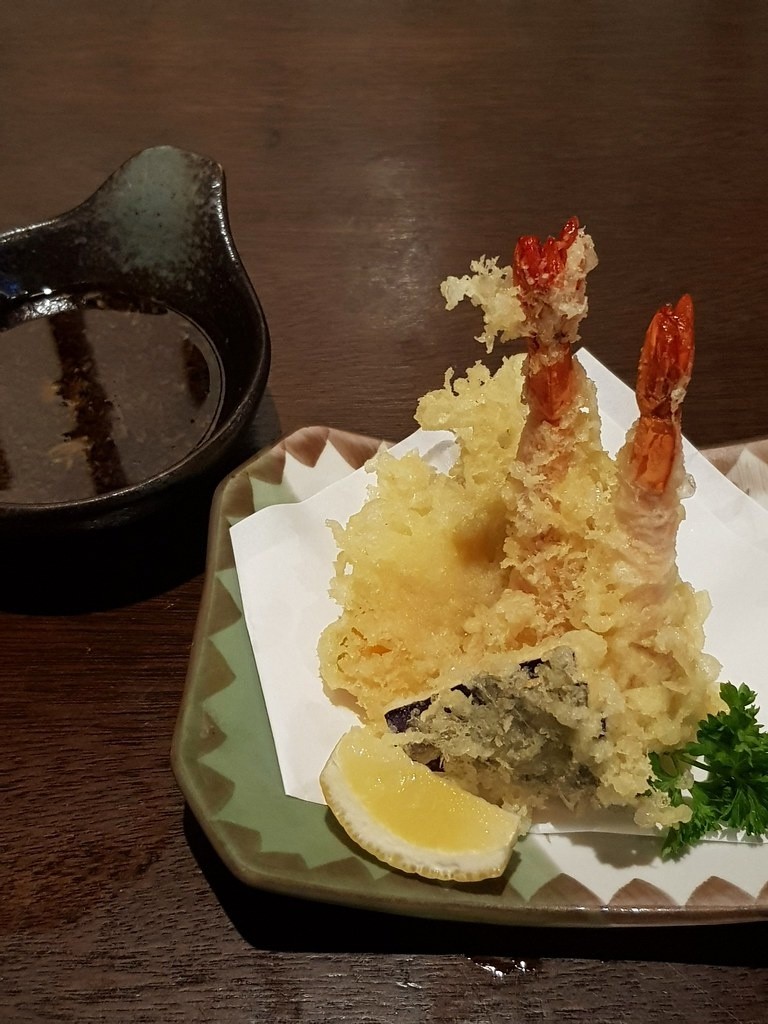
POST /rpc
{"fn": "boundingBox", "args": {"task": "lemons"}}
[321,725,520,882]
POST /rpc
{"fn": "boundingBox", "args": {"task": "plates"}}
[165,422,768,925]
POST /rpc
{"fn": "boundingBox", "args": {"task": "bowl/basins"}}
[0,142,271,547]
